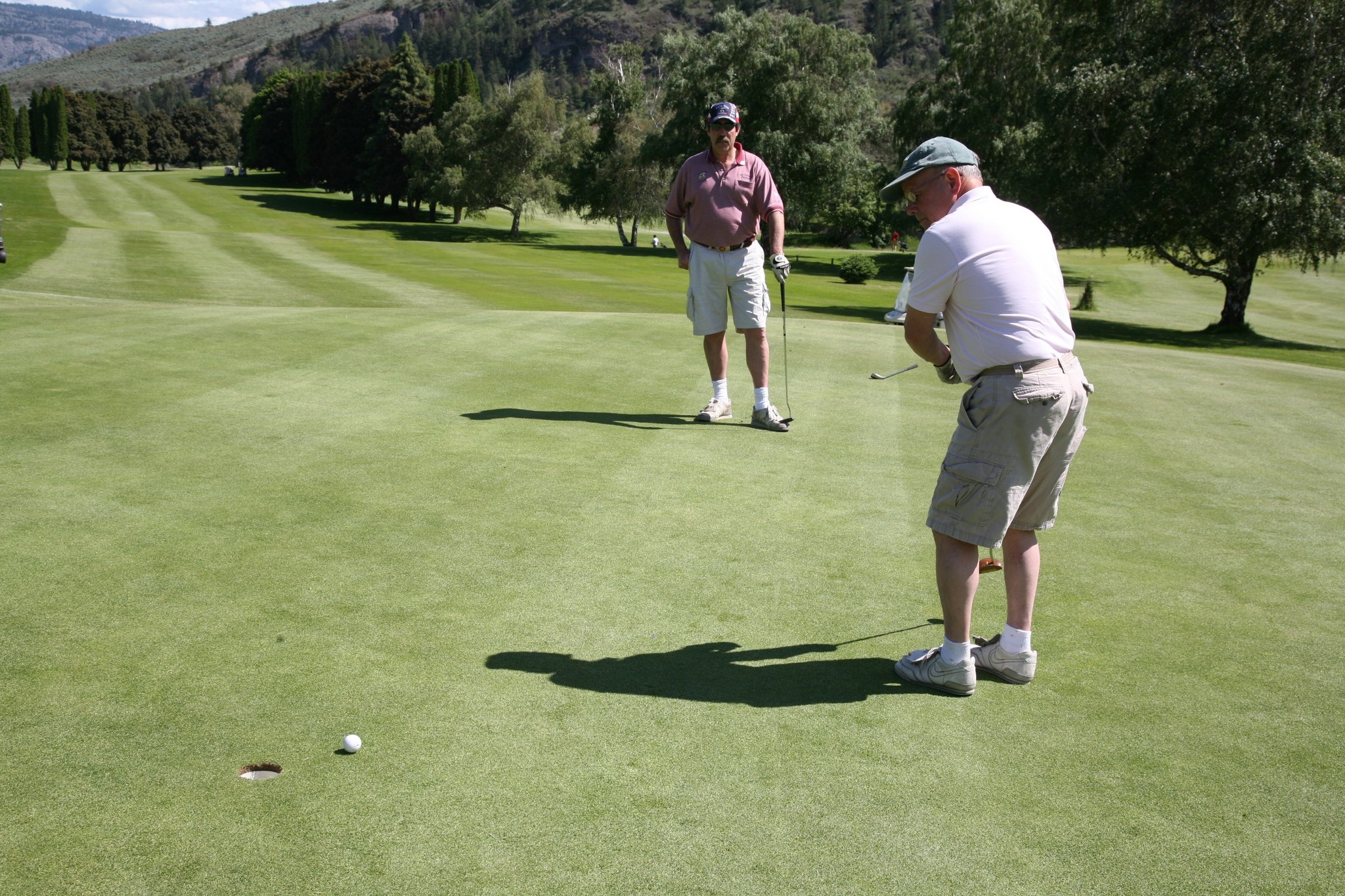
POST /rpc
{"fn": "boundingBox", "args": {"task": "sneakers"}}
[968,634,1037,685]
[895,644,977,694]
[751,406,790,430]
[696,398,732,421]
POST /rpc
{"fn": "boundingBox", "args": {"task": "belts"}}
[978,352,1075,376]
[691,236,757,252]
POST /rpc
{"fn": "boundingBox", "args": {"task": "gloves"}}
[768,253,791,285]
[935,343,962,384]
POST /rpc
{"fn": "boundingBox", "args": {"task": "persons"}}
[890,231,899,252]
[650,235,659,247]
[664,102,791,432]
[879,135,1094,697]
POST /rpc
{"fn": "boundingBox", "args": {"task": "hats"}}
[879,136,978,203]
[707,102,740,124]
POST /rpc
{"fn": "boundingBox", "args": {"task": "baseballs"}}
[343,734,362,753]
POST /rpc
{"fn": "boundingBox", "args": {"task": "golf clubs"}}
[978,548,1004,574]
[870,363,918,380]
[778,281,795,423]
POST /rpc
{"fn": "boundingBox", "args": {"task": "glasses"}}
[709,123,737,131]
[905,172,964,209]
